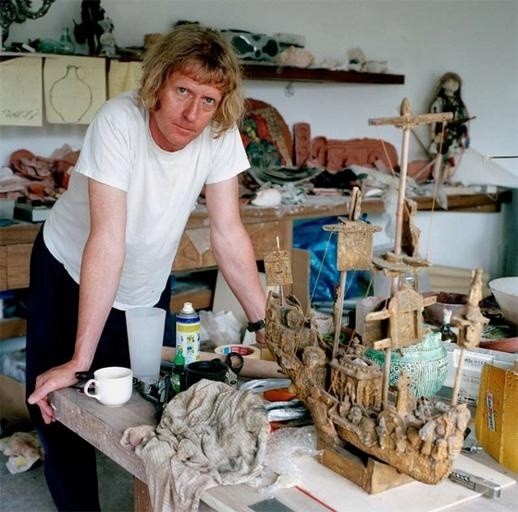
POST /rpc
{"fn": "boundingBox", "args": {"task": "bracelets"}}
[246,320,264,332]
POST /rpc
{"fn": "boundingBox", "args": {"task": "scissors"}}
[211,352,244,390]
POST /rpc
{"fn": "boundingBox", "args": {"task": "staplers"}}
[74,371,95,390]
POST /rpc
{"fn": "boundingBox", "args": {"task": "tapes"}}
[214,344,261,361]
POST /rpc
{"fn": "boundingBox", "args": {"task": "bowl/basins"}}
[424,292,471,324]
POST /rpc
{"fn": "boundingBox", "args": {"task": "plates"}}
[480,338,518,353]
[488,276,518,325]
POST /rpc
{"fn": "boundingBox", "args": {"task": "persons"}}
[26,23,269,512]
[429,72,470,185]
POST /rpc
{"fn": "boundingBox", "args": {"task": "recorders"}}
[218,30,280,61]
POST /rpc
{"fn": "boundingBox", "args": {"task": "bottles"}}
[435,307,457,343]
[172,346,186,394]
[175,301,201,363]
[56,27,74,54]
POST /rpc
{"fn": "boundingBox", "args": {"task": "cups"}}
[84,366,133,408]
[186,360,227,390]
[125,307,166,385]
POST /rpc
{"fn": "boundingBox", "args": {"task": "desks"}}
[285,178,513,316]
[48,326,517,511]
[1,206,283,327]
